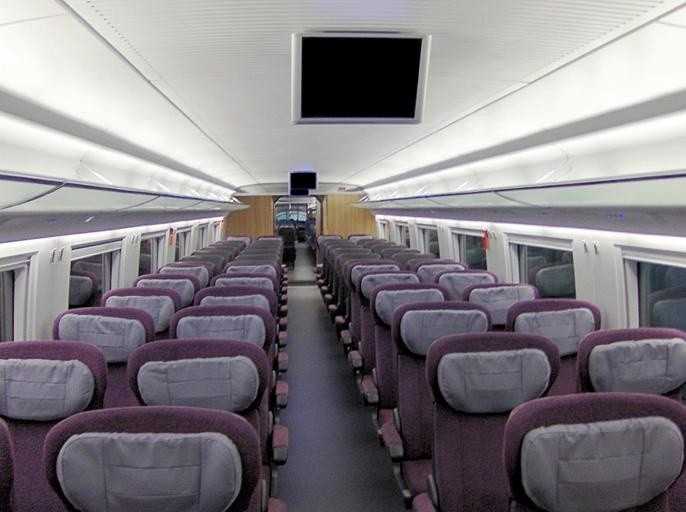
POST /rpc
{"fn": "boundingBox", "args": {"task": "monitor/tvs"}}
[288,189,310,197]
[288,171,319,190]
[290,32,432,127]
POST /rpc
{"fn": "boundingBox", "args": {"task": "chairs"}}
[0,227,302,512]
[309,226,685,511]
